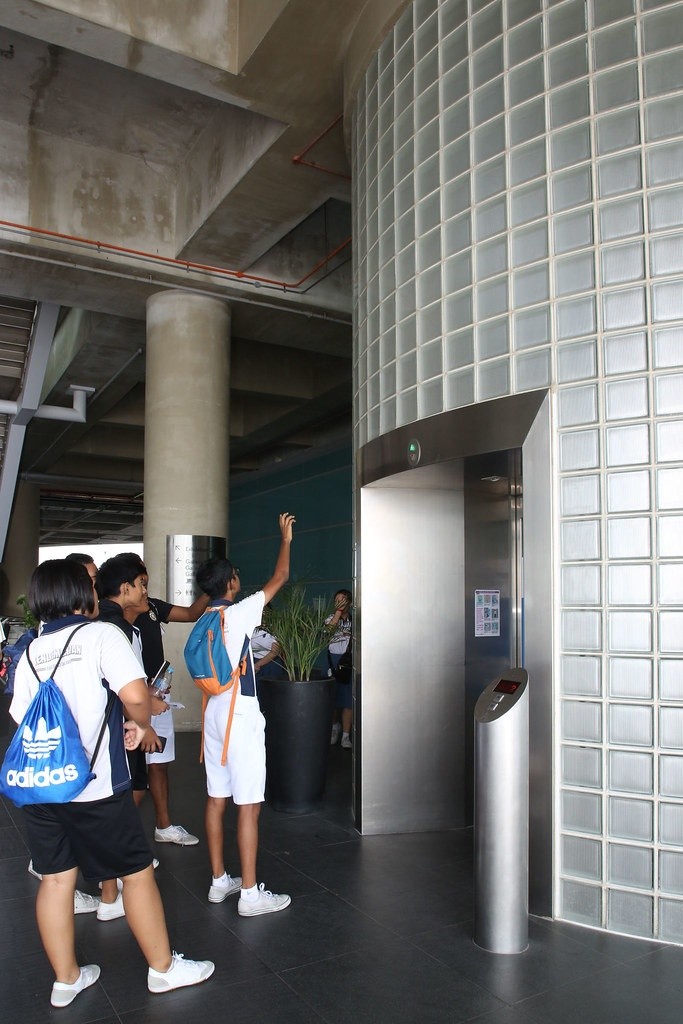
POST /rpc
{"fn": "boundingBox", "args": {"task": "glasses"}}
[224,567,240,588]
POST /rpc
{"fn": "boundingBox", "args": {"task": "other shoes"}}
[330,722,341,744]
[341,737,352,747]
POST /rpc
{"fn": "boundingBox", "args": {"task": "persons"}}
[197,512,296,916]
[28,552,171,921]
[251,629,282,673]
[324,589,353,748]
[8,559,215,1008]
[116,551,208,845]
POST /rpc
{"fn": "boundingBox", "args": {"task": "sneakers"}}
[51,964,101,1008]
[208,874,243,903]
[154,825,199,846]
[96,892,125,921]
[238,883,291,917]
[153,858,159,868]
[28,860,44,880]
[98,876,123,893]
[148,950,215,993]
[73,889,101,914]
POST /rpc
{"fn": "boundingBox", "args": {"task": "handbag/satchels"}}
[332,653,351,686]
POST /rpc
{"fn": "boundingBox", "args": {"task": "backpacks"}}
[0,623,116,807]
[184,605,247,694]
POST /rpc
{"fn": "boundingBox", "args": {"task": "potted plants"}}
[257,572,357,818]
